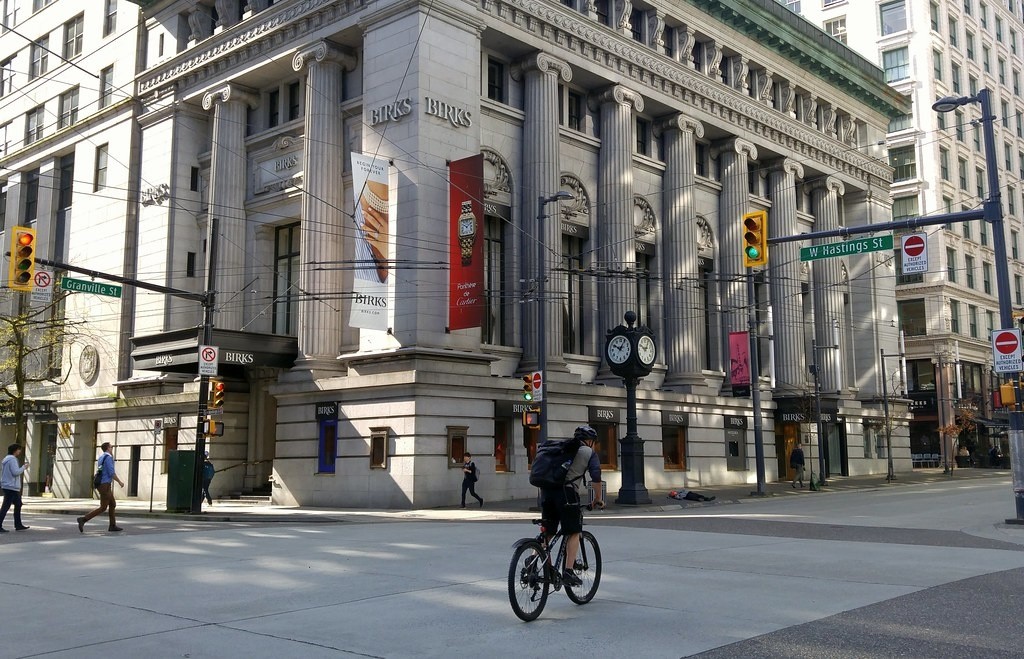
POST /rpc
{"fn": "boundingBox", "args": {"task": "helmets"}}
[574,426,598,440]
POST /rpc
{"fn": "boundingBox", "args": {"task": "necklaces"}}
[363,183,388,214]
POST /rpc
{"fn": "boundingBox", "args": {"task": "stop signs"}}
[904,235,925,257]
[995,331,1019,355]
[533,373,541,390]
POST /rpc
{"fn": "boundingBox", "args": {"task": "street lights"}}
[808,339,838,486]
[880,348,905,480]
[537,190,574,442]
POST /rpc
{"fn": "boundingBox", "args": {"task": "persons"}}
[670,489,715,502]
[525,426,605,585]
[359,179,388,283]
[988,444,999,465]
[459,453,484,509]
[77,442,124,532]
[960,446,975,464]
[0,444,30,532]
[201,451,215,507]
[790,443,806,488]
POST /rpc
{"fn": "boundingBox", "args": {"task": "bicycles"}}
[507,502,603,621]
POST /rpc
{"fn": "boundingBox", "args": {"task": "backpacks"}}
[204,464,214,480]
[470,461,480,482]
[529,439,581,490]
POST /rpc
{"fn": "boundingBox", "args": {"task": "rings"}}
[377,224,380,231]
[374,233,378,238]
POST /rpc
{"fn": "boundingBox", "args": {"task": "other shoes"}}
[801,484,806,488]
[456,506,466,510]
[15,525,30,530]
[792,484,796,488]
[525,556,537,573]
[562,570,582,586]
[207,500,212,504]
[707,497,715,501]
[78,517,83,533]
[1,528,9,533]
[109,526,124,531]
[479,498,484,509]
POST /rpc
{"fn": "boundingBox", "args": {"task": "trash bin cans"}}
[586,480,607,508]
[165,450,194,511]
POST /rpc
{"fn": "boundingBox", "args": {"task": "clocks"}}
[608,336,631,363]
[638,336,655,364]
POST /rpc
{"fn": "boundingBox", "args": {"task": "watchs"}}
[457,200,477,266]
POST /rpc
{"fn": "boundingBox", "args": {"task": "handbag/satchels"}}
[93,455,109,488]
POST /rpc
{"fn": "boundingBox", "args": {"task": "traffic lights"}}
[522,373,534,403]
[213,381,225,408]
[203,419,209,434]
[742,211,768,267]
[525,410,539,427]
[7,226,36,292]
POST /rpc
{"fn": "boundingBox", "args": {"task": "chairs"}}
[912,454,939,468]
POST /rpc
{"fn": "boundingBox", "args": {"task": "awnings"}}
[956,414,1009,428]
